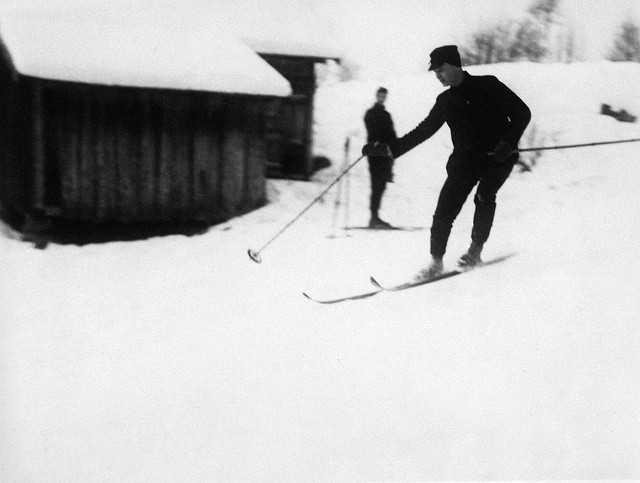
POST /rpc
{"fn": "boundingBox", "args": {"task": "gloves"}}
[362,141,392,160]
[492,140,513,164]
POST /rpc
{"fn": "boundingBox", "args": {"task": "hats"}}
[428,44,462,72]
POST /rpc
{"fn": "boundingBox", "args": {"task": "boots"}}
[418,253,445,278]
[458,238,483,266]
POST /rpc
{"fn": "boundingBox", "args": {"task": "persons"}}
[363,44,531,283]
[364,87,398,230]
